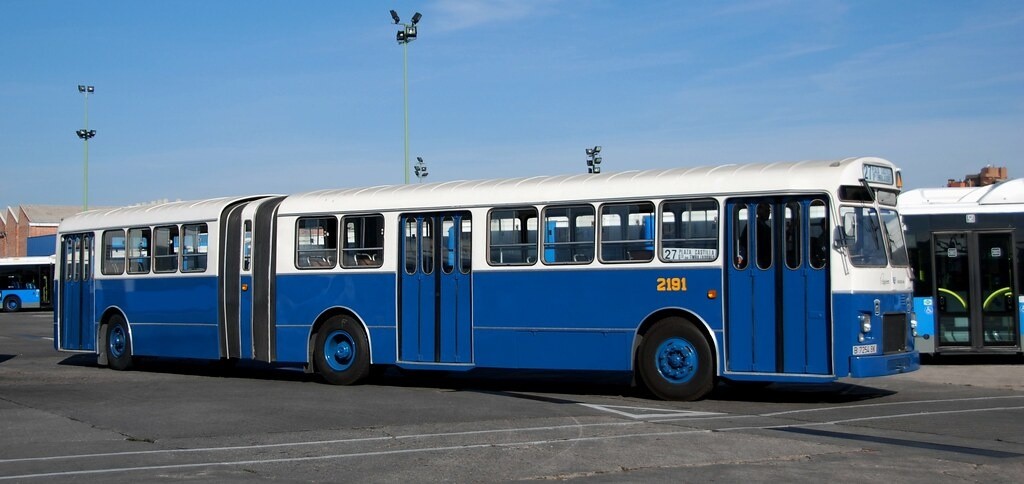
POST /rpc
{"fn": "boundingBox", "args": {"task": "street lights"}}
[74,84,97,211]
[389,9,422,185]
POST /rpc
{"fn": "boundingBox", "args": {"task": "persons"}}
[740,203,771,268]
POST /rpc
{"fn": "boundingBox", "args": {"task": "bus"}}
[0,156,1023,404]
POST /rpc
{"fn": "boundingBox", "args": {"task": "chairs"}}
[307,256,334,266]
[354,253,382,266]
[12,281,37,289]
[104,262,143,273]
